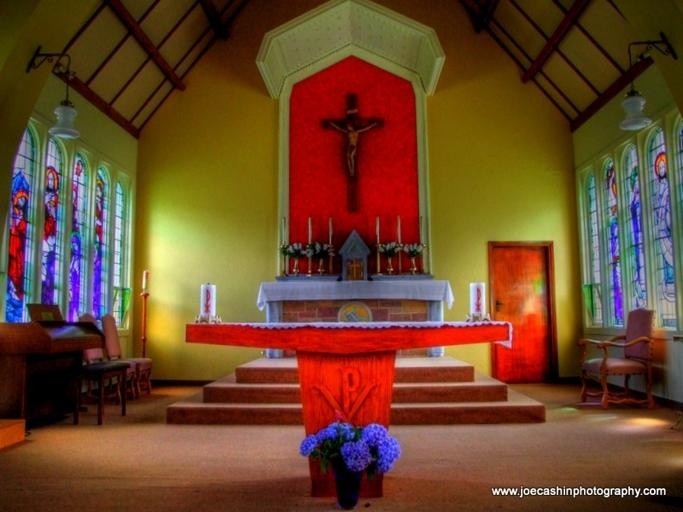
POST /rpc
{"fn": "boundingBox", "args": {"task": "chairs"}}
[78,312,154,426]
[576,308,657,411]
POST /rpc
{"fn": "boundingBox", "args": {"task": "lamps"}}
[617,30,678,132]
[23,45,78,144]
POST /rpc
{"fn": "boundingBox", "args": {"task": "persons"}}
[6,190,28,324]
[655,152,677,302]
[42,165,59,250]
[331,122,376,177]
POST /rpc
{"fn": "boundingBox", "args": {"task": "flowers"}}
[298,420,400,476]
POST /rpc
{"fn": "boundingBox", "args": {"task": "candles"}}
[308,216,311,244]
[397,215,400,242]
[420,215,423,242]
[469,281,486,316]
[199,283,217,319]
[142,270,149,289]
[281,216,286,241]
[329,218,332,245]
[375,216,380,242]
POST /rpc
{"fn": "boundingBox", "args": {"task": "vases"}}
[330,456,363,511]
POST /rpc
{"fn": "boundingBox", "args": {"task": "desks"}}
[188,319,515,497]
[256,277,455,359]
